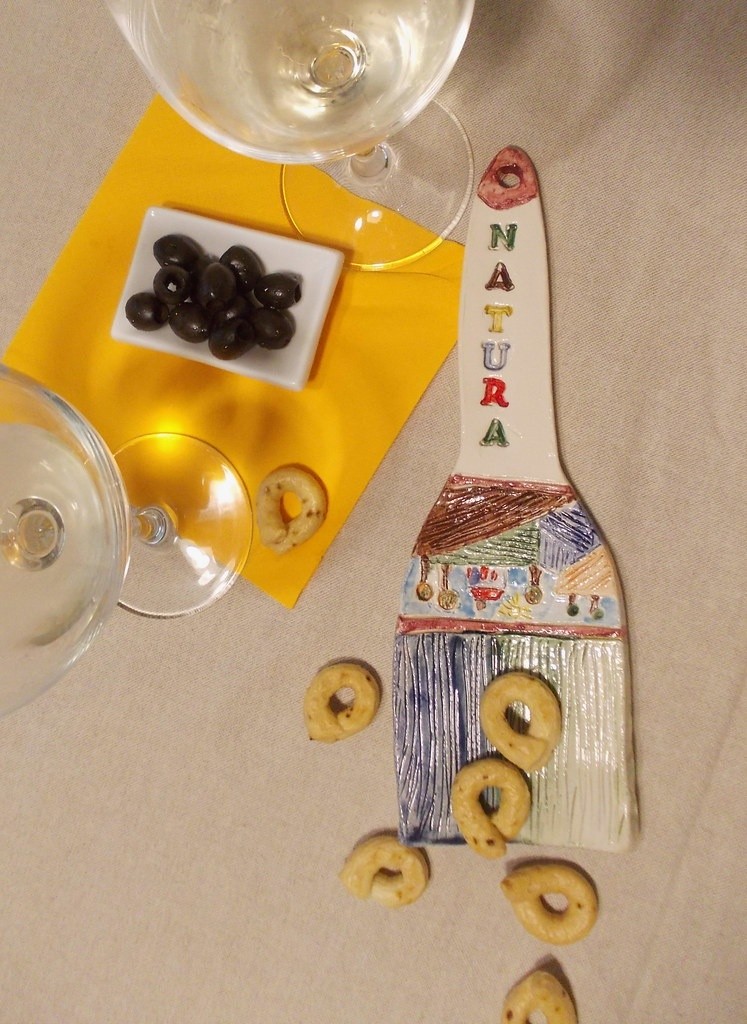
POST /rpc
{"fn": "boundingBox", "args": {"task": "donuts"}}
[253,463,598,1024]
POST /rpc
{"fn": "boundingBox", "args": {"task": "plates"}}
[110,206,344,392]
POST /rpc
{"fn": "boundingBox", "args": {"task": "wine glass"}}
[1,363,251,715]
[100,1,474,269]
